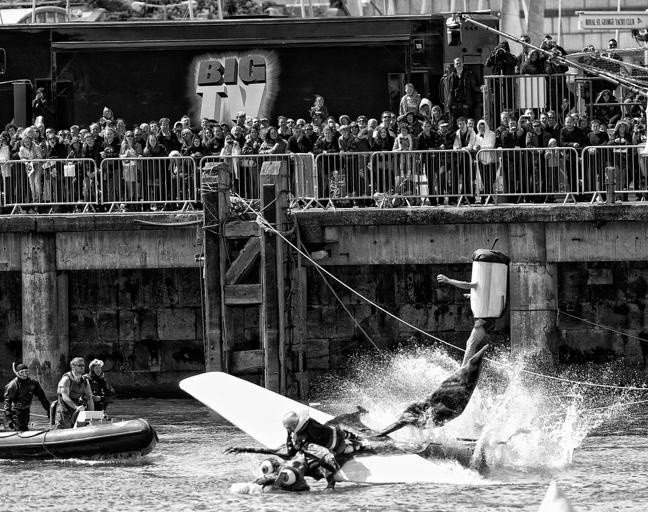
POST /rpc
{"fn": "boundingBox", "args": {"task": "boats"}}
[178,366,460,489]
[1,416,160,460]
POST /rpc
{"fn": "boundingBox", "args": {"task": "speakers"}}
[55,80,74,128]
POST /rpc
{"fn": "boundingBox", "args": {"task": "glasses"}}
[74,364,86,367]
[17,370,29,376]
[126,130,141,138]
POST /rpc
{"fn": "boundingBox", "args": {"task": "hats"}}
[89,358,103,368]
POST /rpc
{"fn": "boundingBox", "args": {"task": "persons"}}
[54,357,94,429]
[82,359,115,412]
[0,29,647,215]
[436,237,509,367]
[3,364,50,431]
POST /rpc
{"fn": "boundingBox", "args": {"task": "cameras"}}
[496,49,506,56]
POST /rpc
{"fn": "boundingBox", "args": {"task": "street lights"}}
[130,1,198,21]
